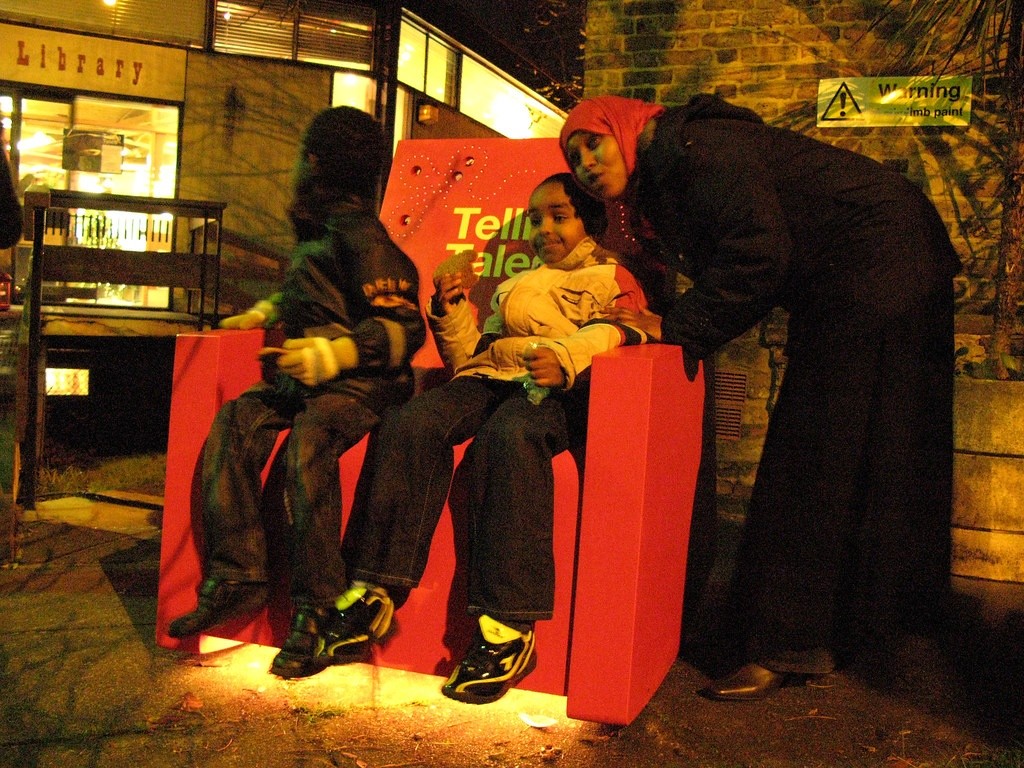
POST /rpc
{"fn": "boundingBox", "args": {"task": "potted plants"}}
[846,0,1024,583]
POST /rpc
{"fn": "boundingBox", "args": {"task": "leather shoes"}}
[710,661,788,700]
[167,582,274,638]
[271,609,347,677]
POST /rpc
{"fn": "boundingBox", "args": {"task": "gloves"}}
[218,299,281,329]
[276,336,359,386]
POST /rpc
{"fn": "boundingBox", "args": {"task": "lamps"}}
[416,98,439,124]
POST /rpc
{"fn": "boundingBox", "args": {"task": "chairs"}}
[156,137,719,724]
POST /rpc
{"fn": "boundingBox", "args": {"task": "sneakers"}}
[316,584,395,655]
[441,616,539,700]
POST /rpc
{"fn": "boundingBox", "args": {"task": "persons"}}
[168,107,426,681]
[310,173,653,704]
[558,94,964,701]
[0,125,23,250]
